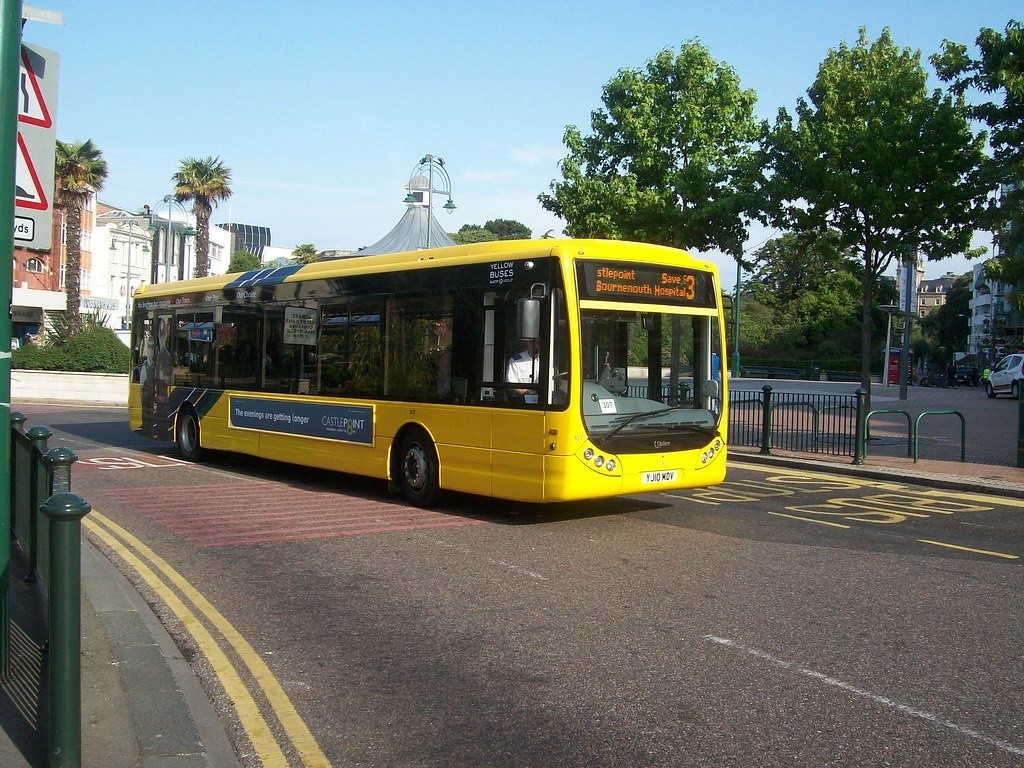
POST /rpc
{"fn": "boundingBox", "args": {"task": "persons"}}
[505,339,541,398]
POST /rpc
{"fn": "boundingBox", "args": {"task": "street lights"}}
[146,198,199,284]
[959,315,971,364]
[405,155,459,249]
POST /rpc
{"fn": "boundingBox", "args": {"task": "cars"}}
[984,352,1024,399]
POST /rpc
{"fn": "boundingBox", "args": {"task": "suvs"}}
[956,366,980,387]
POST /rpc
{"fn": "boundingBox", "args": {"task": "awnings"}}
[179,321,216,331]
[321,315,380,325]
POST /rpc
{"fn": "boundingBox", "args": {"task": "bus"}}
[126,238,731,504]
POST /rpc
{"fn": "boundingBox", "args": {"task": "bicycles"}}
[920,370,961,389]
